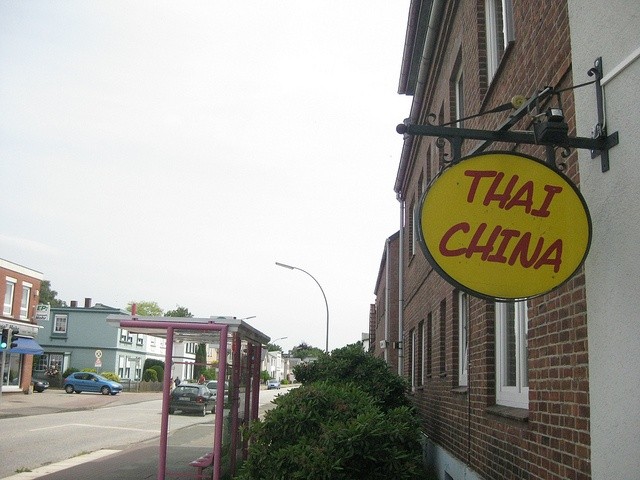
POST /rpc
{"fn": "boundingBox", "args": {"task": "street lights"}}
[276,262,328,357]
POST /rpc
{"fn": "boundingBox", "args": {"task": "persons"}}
[198,373,205,384]
[169,377,180,391]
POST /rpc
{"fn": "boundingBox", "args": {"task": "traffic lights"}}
[0,329,8,349]
[10,328,19,349]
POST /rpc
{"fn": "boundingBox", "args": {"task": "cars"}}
[64,372,123,395]
[169,384,216,416]
[206,381,240,409]
[12,378,49,392]
[268,379,281,390]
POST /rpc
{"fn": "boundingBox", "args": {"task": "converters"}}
[545,105,565,122]
